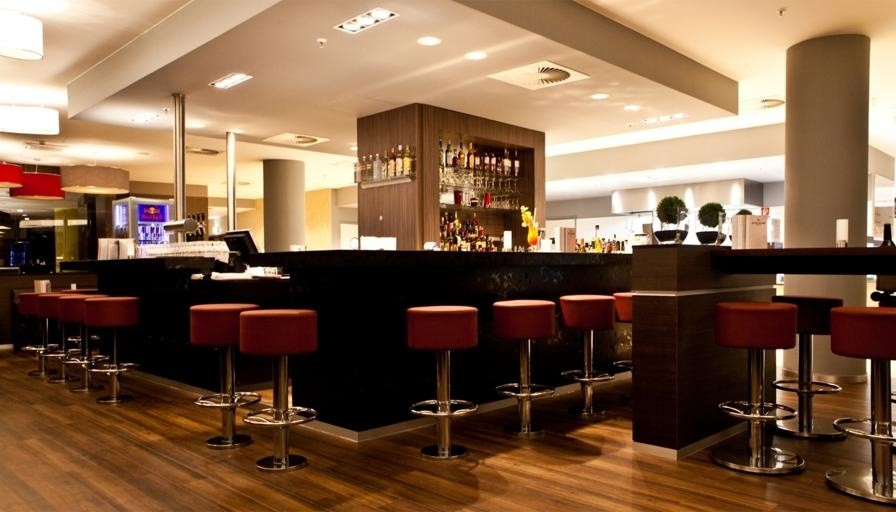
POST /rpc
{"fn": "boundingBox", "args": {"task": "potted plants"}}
[650,196,754,247]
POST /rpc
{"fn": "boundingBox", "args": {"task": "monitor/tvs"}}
[224,230,260,256]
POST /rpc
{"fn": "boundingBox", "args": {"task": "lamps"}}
[1,14,134,203]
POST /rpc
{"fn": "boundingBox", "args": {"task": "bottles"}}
[437,167,473,207]
[437,139,522,177]
[354,143,415,183]
[875,222,895,293]
[574,223,626,253]
[113,223,130,238]
[136,223,164,244]
[439,210,494,253]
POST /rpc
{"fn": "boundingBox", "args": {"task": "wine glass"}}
[472,174,520,210]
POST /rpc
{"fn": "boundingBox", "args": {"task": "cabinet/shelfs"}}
[437,163,522,216]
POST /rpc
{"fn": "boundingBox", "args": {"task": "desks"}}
[631,243,896,469]
